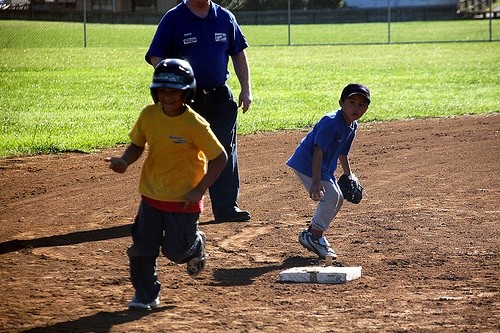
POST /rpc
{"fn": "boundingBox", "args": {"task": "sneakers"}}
[186,231,208,276]
[128,291,161,310]
[298,230,338,260]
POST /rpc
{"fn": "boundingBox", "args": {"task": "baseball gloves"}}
[338,174,363,204]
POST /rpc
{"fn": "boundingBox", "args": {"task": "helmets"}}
[149,58,197,105]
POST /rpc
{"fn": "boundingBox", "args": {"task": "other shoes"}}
[214,204,251,223]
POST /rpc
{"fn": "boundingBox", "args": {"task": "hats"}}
[341,83,371,105]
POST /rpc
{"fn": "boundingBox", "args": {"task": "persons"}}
[102,59,227,308]
[285,84,371,259]
[144,0,252,222]
